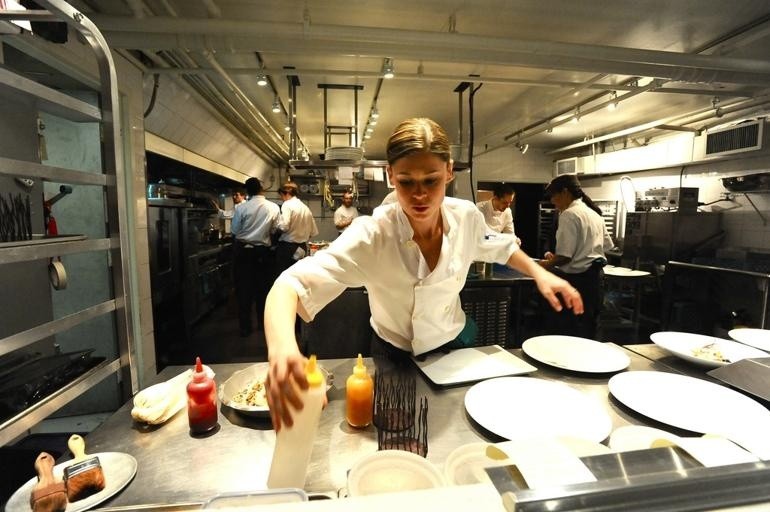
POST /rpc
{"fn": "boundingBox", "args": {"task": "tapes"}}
[49,261,67,290]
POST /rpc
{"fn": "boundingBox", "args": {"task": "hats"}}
[542,175,580,196]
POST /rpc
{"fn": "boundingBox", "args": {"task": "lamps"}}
[382,60,395,80]
[257,62,311,162]
[516,143,528,155]
[547,100,618,134]
[362,102,379,163]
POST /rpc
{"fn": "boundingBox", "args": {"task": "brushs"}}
[30,451,65,512]
[64,434,105,502]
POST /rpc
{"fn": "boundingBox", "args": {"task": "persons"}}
[543,209,558,260]
[539,175,615,342]
[333,191,359,235]
[476,185,521,246]
[262,118,585,433]
[209,178,319,337]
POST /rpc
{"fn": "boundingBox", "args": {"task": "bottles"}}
[186,357,220,432]
[266,356,326,491]
[345,353,374,429]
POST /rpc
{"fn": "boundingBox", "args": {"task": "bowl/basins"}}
[217,362,334,416]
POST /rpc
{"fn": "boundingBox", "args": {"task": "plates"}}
[650,330,770,367]
[464,377,612,444]
[409,343,538,387]
[324,146,364,161]
[4,451,138,512]
[608,370,770,435]
[608,425,681,452]
[728,327,770,353]
[521,335,631,375]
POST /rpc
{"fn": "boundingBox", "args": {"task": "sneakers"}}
[240,328,252,337]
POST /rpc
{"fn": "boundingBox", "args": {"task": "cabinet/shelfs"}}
[537,200,620,254]
